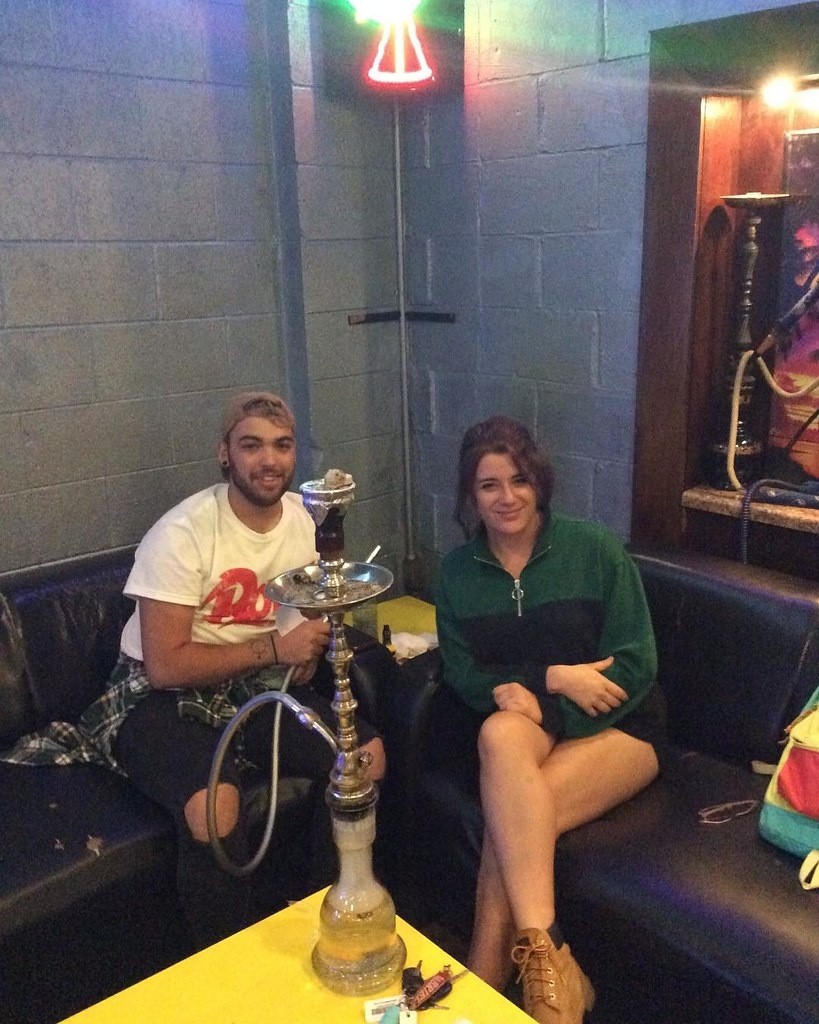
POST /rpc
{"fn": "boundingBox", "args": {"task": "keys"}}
[379,959,471,1024]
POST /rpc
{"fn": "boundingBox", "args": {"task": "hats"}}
[216,391,284,480]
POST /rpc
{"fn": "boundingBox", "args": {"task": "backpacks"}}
[750,688,819,890]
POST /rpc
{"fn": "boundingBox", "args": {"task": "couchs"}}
[0,536,819,1024]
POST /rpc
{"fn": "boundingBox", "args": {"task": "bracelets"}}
[269,633,278,666]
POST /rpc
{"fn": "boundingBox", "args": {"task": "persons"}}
[89,390,386,950]
[430,414,676,1022]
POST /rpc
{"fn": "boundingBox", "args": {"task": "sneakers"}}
[512,928,596,1024]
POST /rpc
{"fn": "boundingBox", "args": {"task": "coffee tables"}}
[60,885,545,1024]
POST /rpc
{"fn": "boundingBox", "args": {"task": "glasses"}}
[698,799,758,824]
